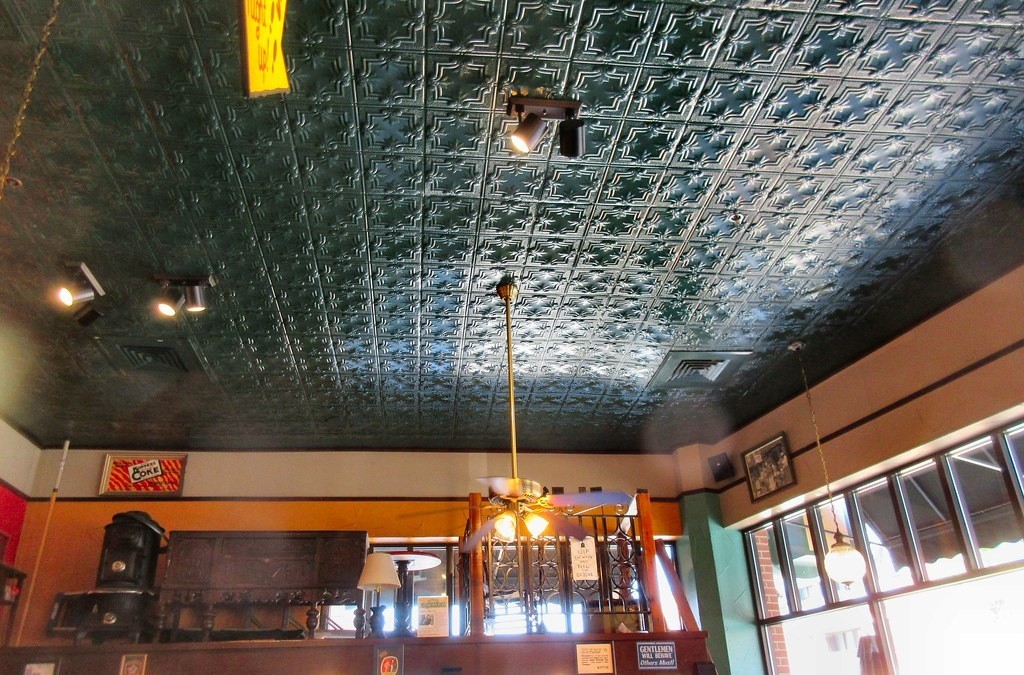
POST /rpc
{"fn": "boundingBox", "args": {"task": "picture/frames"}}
[741,431,797,503]
[98,451,188,497]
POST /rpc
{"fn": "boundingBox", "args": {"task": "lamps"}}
[58,262,106,326]
[357,553,401,639]
[504,96,586,158]
[787,340,867,589]
[154,274,220,316]
[493,506,548,539]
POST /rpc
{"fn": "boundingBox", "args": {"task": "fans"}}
[396,277,633,552]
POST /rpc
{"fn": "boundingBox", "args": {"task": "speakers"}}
[707,452,735,482]
